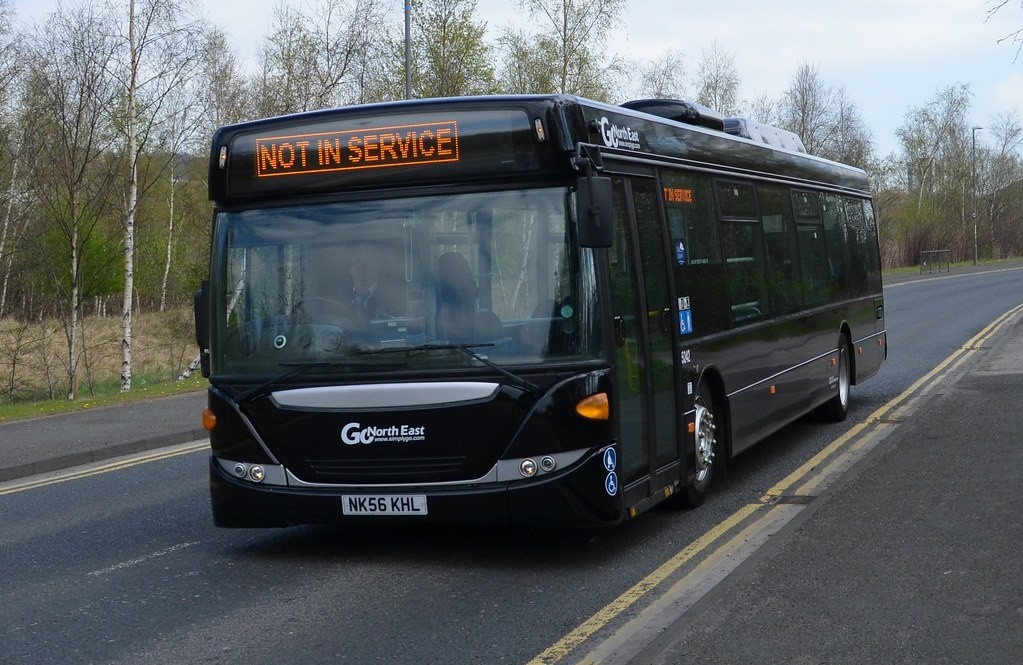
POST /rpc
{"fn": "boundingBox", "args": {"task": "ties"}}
[358,292,368,308]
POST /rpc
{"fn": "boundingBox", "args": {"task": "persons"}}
[321,243,395,352]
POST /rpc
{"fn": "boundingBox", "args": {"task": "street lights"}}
[971,125,984,265]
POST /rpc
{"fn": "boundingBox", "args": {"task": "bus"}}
[191,92,888,533]
